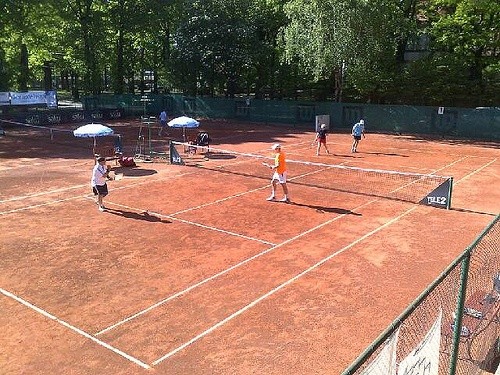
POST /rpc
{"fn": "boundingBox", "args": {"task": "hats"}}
[97,158,105,161]
[321,124,326,128]
[271,144,280,149]
[360,120,364,124]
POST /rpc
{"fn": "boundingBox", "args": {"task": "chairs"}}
[190,132,209,153]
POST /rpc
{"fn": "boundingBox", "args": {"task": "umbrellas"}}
[73,123,114,155]
[166,115,200,142]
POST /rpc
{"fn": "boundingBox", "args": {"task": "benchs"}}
[92,146,121,166]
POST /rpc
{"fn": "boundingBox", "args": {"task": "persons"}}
[158,109,172,137]
[266,144,290,202]
[91,158,113,211]
[351,120,365,152]
[488,99,496,106]
[315,124,329,156]
[114,135,123,166]
[246,97,250,106]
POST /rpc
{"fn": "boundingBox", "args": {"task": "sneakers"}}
[280,198,288,202]
[100,208,106,212]
[267,197,276,201]
